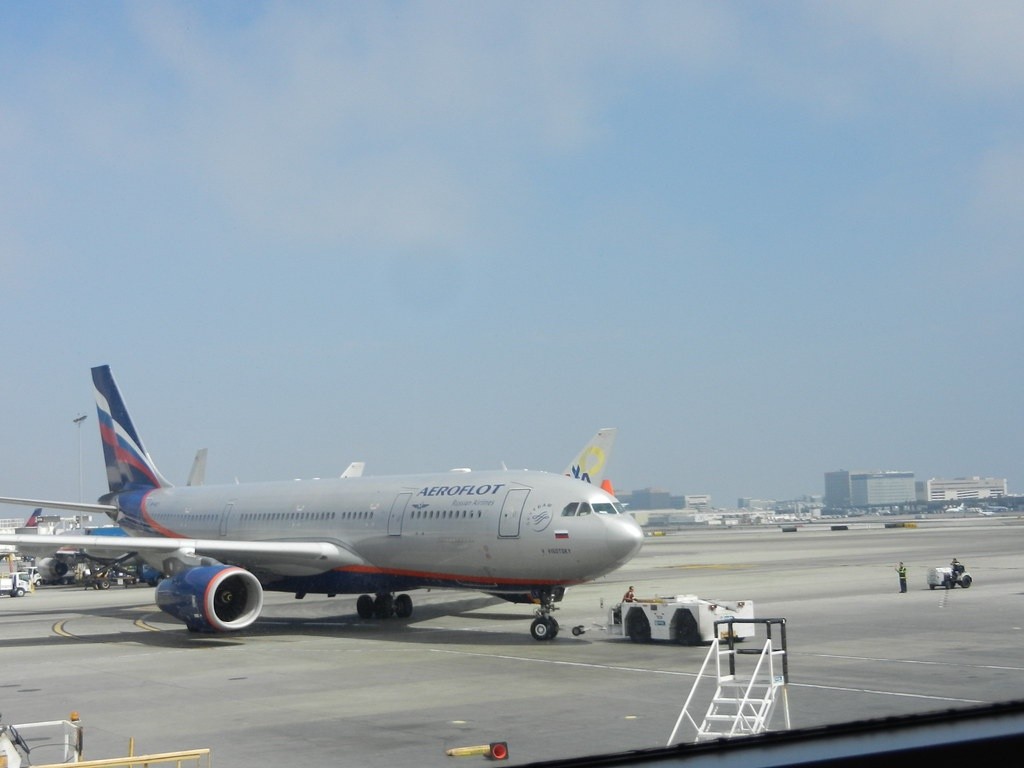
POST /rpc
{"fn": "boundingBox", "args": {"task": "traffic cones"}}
[485,740,510,761]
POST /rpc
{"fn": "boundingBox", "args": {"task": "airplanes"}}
[1,365,646,643]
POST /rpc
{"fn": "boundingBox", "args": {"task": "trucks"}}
[0,572,31,596]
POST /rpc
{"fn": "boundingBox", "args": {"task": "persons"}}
[623,586,638,603]
[895,562,907,594]
